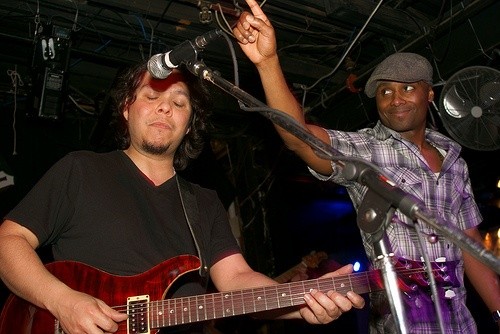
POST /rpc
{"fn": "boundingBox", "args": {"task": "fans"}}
[440,66,500,150]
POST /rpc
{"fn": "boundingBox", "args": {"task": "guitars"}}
[0,253,461,334]
[274,250,329,284]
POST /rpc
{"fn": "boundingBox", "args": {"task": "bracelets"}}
[491,309,500,323]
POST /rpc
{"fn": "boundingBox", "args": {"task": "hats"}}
[364,52,432,97]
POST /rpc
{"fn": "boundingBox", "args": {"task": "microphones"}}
[146,28,220,81]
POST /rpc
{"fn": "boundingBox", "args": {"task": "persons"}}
[0,58,365,334]
[232,0,500,334]
